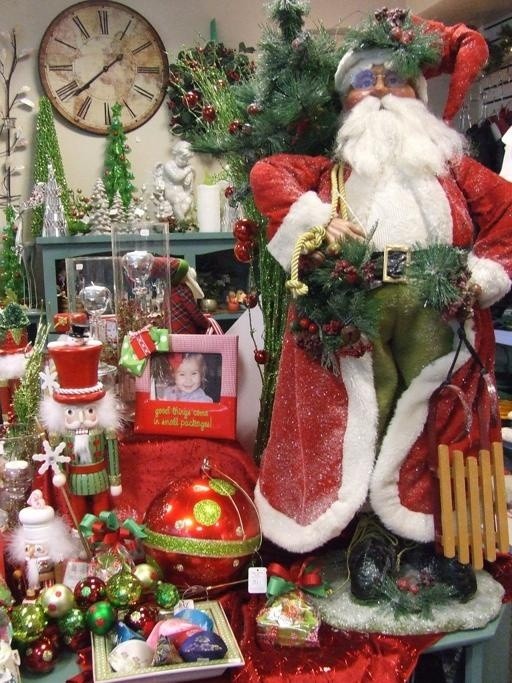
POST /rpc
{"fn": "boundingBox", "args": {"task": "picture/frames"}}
[133,333,237,440]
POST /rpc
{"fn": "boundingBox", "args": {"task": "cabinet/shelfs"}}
[31,231,245,343]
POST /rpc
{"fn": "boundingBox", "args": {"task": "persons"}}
[244,9,508,570]
[159,353,215,403]
[45,339,123,523]
[163,140,199,228]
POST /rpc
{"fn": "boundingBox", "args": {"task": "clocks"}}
[37,0,169,136]
